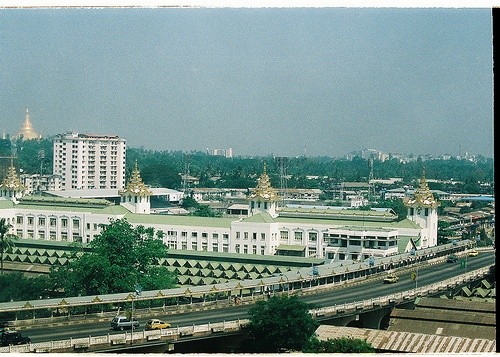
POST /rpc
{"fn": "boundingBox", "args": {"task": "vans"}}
[111,316,140,331]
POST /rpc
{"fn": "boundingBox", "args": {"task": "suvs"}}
[1,329,31,347]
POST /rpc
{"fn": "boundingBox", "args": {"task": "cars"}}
[447,255,459,263]
[468,250,479,257]
[144,319,171,331]
[383,275,400,284]
